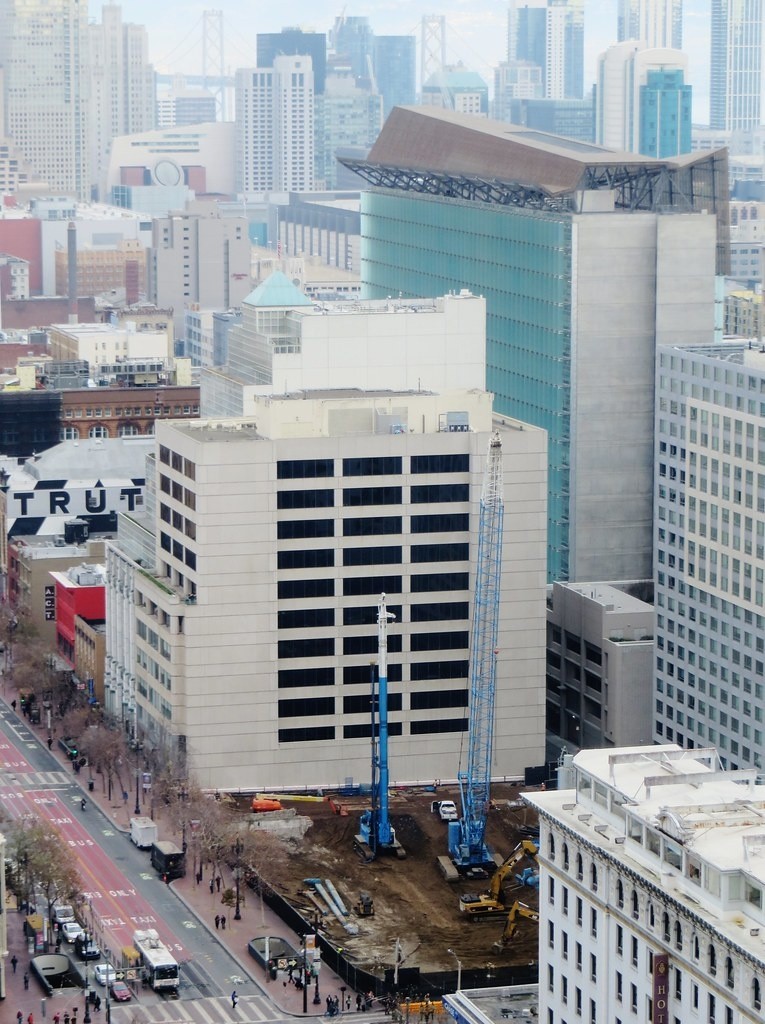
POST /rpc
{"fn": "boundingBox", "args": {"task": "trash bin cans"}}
[89,782,94,791]
[71,760,81,774]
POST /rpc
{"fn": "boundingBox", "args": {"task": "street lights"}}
[447,949,462,990]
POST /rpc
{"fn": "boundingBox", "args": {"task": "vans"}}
[50,898,76,930]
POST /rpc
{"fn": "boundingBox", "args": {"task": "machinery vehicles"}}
[352,591,407,860]
[487,899,540,957]
[433,428,507,880]
[356,892,374,917]
[252,792,348,817]
[457,839,538,921]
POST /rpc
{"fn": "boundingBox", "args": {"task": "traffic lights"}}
[21,695,25,704]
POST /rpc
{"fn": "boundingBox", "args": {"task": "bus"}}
[131,928,181,990]
[151,839,186,878]
[130,817,158,849]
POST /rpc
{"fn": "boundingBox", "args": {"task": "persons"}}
[24,972,29,990]
[81,799,86,810]
[289,968,310,990]
[17,1009,22,1024]
[11,955,18,973]
[232,991,238,1008]
[92,995,101,1013]
[326,992,430,1014]
[56,936,61,946]
[11,700,16,711]
[215,915,226,930]
[47,738,53,750]
[209,876,221,894]
[53,1011,77,1024]
[28,1013,33,1024]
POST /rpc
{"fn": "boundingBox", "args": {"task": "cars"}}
[74,934,101,960]
[62,922,84,943]
[95,964,117,986]
[431,799,459,821]
[110,982,132,1002]
